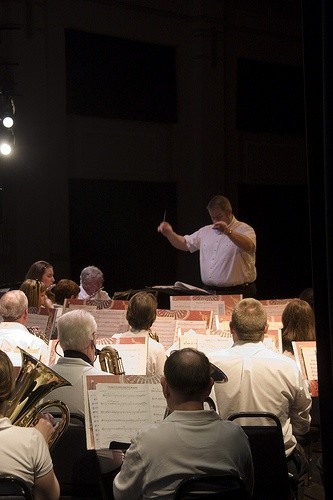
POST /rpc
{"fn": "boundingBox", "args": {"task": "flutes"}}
[47,284,56,291]
[89,287,104,298]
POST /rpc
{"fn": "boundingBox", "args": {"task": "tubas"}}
[95,346,126,375]
[4,345,74,451]
[163,349,228,420]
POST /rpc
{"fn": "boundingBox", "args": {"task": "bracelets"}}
[227,229,232,236]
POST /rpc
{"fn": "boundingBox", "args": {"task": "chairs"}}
[50,412,109,500]
[0,475,33,500]
[172,412,290,500]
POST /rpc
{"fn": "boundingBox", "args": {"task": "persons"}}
[25,261,55,290]
[158,196,257,301]
[281,300,316,361]
[37,310,125,474]
[78,266,111,300]
[0,349,60,500]
[53,279,81,308]
[0,290,48,367]
[208,298,312,500]
[113,348,254,500]
[20,279,54,309]
[112,291,166,376]
